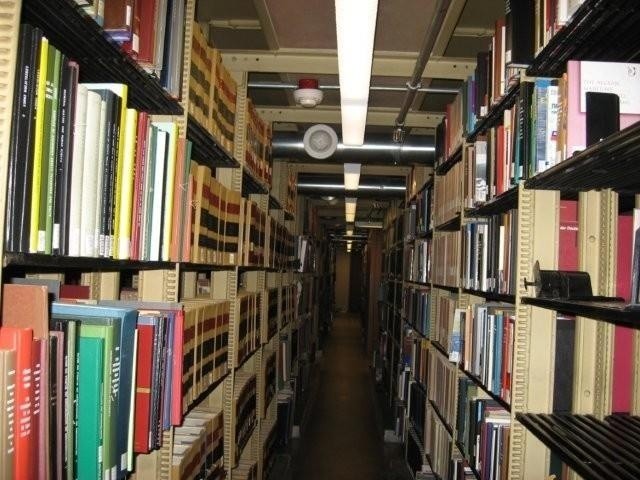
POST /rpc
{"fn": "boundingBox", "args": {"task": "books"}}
[0,1,329,479]
[374,0,640,480]
